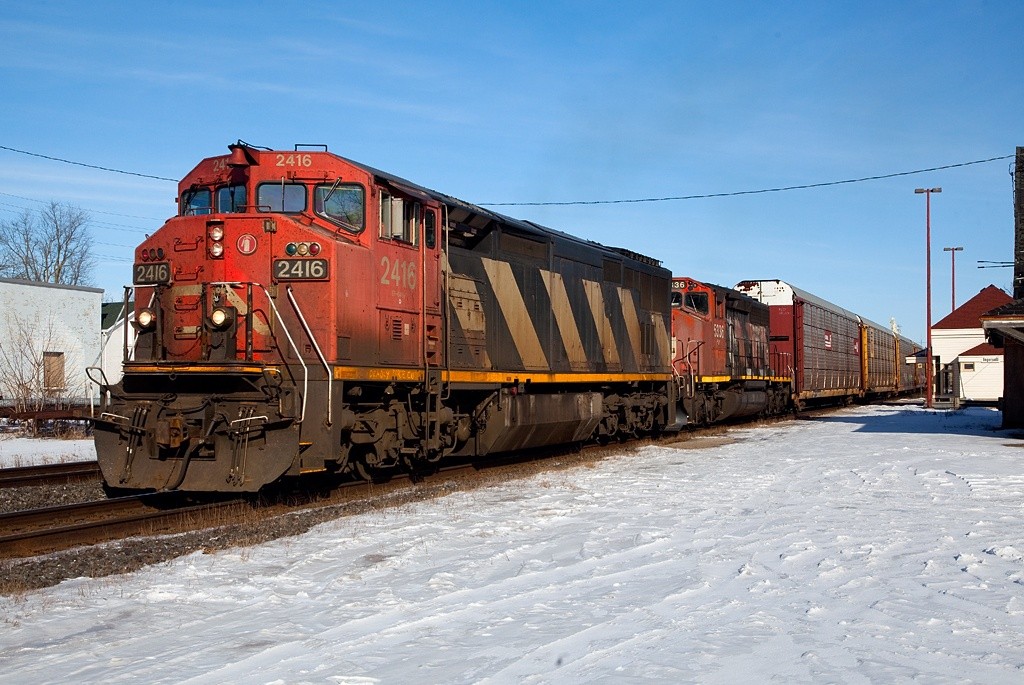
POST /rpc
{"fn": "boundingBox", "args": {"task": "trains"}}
[84,139,932,494]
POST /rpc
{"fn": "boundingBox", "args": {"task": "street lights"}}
[943,247,963,313]
[915,188,944,407]
